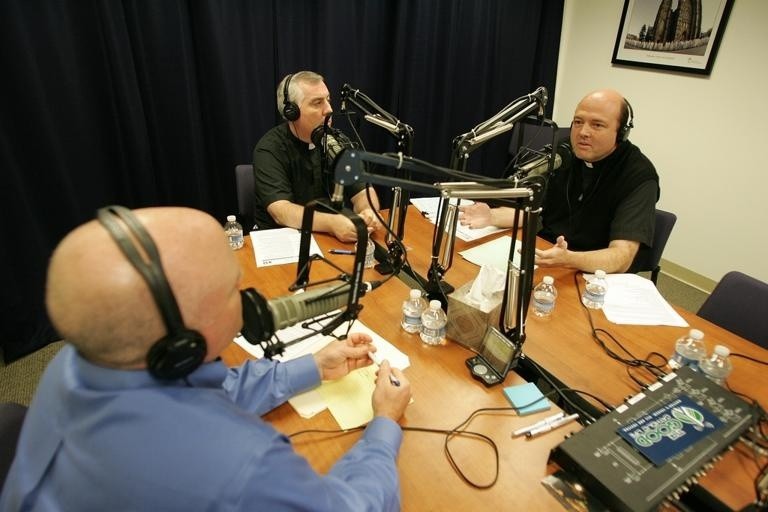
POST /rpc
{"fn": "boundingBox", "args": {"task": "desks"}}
[213,225,691,512]
[369,199,767,511]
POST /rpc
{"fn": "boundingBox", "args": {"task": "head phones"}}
[97,205,207,379]
[570,98,634,145]
[283,74,300,121]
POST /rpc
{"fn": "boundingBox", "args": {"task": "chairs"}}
[695,269,768,351]
[632,209,677,286]
[0,399,33,493]
[508,121,572,159]
[232,162,258,231]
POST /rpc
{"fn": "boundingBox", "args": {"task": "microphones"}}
[240,280,382,346]
[310,125,342,161]
[515,143,574,176]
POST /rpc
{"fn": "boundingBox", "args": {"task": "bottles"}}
[222,215,244,251]
[354,233,375,268]
[580,268,609,310]
[667,328,734,387]
[532,277,557,318]
[399,288,449,347]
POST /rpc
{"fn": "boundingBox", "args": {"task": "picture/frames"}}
[608,1,733,75]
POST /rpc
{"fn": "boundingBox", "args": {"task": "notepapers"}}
[503,381,550,416]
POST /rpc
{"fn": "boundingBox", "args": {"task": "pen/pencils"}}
[367,352,401,387]
[328,249,356,255]
[512,412,578,438]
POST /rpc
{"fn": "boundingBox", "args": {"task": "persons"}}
[249,69,385,245]
[0,204,414,511]
[454,86,661,278]
